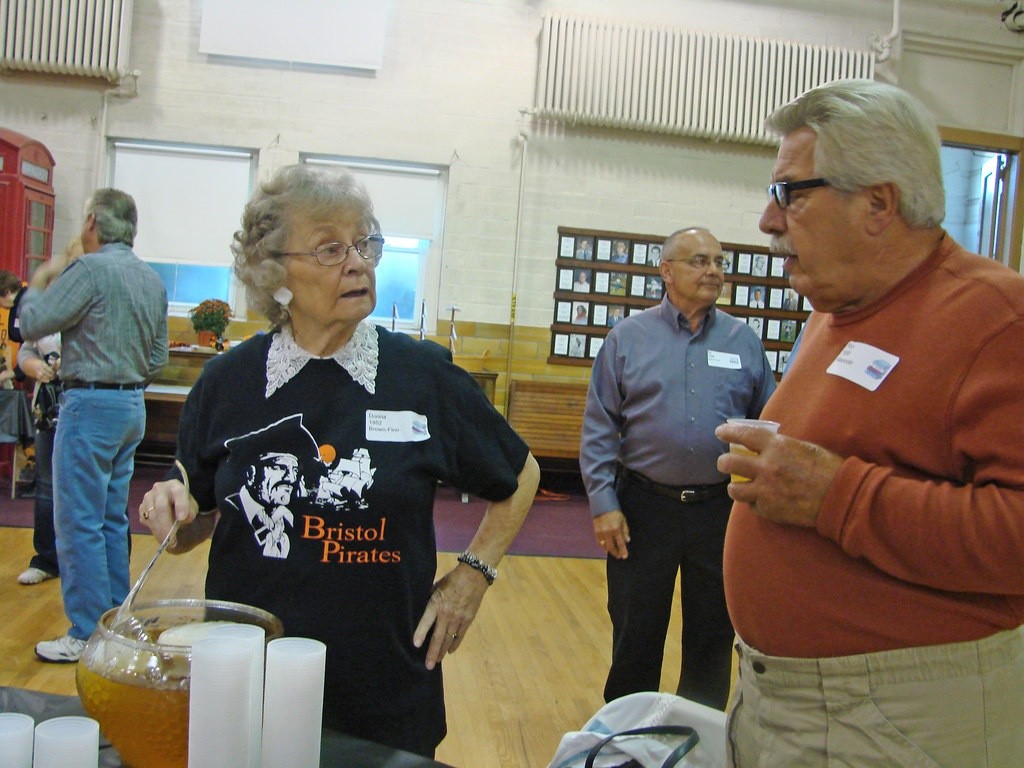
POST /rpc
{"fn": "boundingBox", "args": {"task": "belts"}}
[619,465,728,504]
[63,379,145,390]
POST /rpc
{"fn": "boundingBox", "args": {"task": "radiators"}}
[0,0,134,83]
[520,14,876,148]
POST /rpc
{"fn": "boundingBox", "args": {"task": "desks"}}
[0,685,457,768]
[169,344,224,358]
[134,383,193,466]
[0,387,37,498]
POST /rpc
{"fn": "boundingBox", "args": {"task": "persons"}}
[574,268,589,293]
[573,305,587,324]
[0,187,168,664]
[615,274,621,289]
[577,226,777,712]
[650,277,660,296]
[647,245,662,267]
[576,237,592,261]
[570,337,583,357]
[608,305,624,328]
[612,240,628,263]
[139,161,541,762]
[715,79,1024,768]
[720,254,799,373]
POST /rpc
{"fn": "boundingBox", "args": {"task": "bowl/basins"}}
[76,598,284,768]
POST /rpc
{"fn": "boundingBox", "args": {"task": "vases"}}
[198,329,219,347]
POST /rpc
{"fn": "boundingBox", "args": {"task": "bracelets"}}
[457,550,498,583]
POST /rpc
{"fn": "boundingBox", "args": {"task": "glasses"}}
[667,256,731,269]
[767,177,830,208]
[272,233,385,267]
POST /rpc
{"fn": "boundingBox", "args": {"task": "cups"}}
[726,417,780,483]
[0,712,100,768]
[187,622,328,768]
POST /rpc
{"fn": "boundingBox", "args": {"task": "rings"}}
[143,506,154,518]
[447,631,458,639]
[600,540,605,545]
[38,371,43,376]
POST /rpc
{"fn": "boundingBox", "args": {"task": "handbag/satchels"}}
[33,352,62,432]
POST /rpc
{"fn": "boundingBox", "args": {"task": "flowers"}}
[189,298,234,337]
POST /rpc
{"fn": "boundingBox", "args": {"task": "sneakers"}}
[17,567,51,583]
[35,634,87,662]
